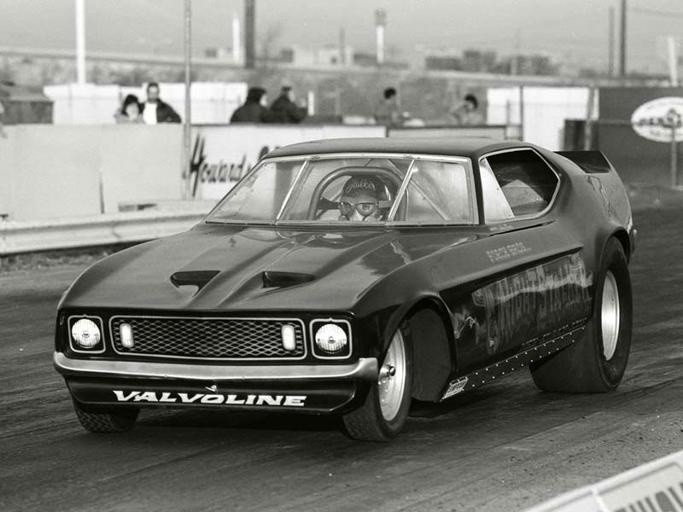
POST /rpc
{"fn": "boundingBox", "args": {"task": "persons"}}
[113,94,145,125]
[271,85,308,124]
[230,86,275,123]
[451,94,483,126]
[338,176,390,222]
[375,88,404,128]
[140,82,181,123]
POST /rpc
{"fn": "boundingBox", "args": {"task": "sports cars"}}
[53,131,642,440]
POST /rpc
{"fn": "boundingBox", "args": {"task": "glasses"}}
[339,199,378,217]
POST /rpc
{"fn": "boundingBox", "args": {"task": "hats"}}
[338,173,390,206]
